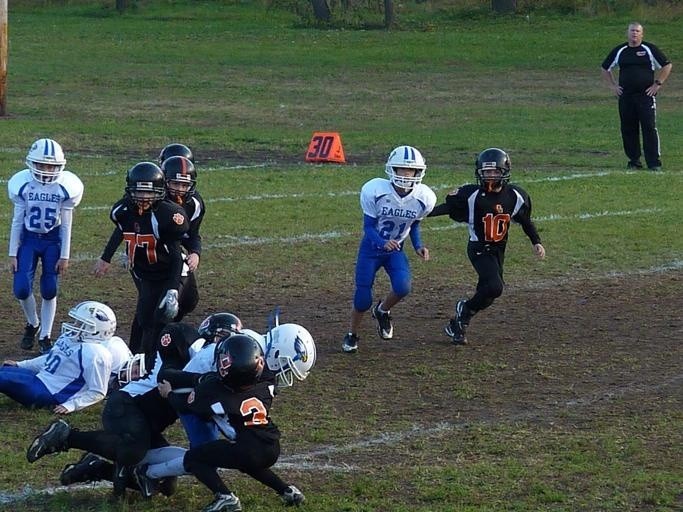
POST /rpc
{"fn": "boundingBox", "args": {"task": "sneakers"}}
[627,160,642,170]
[21,321,42,350]
[455,298,470,330]
[26,416,306,512]
[370,299,394,340]
[444,318,468,345]
[38,336,53,354]
[341,331,360,353]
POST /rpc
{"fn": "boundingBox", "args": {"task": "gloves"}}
[158,289,179,319]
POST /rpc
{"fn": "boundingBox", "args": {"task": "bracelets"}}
[655,79,663,86]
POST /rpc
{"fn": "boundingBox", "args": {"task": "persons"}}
[109,162,193,380]
[5,137,85,354]
[0,301,117,416]
[427,148,546,347]
[341,145,438,353]
[90,156,206,323]
[601,22,673,173]
[27,312,318,512]
[158,143,196,168]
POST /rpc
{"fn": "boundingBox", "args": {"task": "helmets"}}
[124,143,199,213]
[385,145,427,189]
[60,300,117,344]
[474,147,512,181]
[198,312,317,388]
[25,138,66,185]
